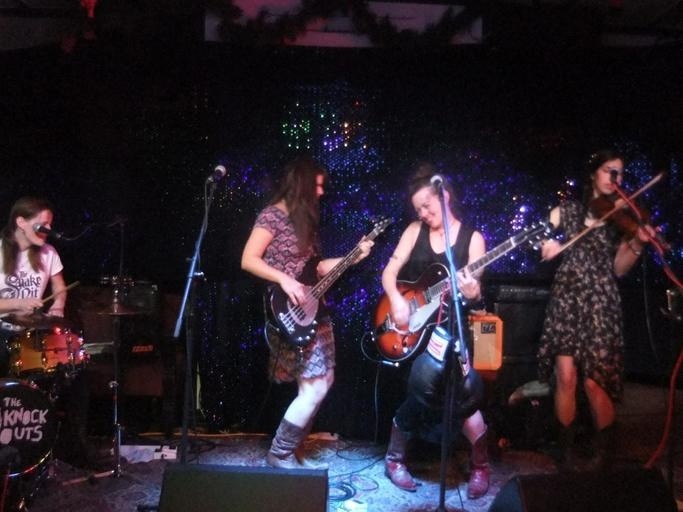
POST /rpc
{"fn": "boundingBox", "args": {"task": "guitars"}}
[371,215,549,361]
[266,216,396,348]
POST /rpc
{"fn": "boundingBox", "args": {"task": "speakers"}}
[488,467,679,512]
[62,282,185,400]
[159,463,329,511]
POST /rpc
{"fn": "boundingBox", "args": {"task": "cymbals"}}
[1,312,71,328]
[89,281,156,316]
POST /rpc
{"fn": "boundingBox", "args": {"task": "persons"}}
[381,162,492,499]
[537,149,657,476]
[240,158,375,471]
[0,195,117,471]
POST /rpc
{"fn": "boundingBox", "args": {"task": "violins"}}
[592,195,683,288]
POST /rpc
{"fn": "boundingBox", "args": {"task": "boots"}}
[461,423,491,498]
[265,417,329,470]
[596,419,653,465]
[551,415,579,473]
[385,417,418,491]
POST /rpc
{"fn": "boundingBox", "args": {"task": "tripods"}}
[60,211,146,485]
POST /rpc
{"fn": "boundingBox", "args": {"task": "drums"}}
[0,377,63,495]
[7,324,82,377]
[71,312,115,349]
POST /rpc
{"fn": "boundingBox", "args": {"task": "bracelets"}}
[629,244,643,256]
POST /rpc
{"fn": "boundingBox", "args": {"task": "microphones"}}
[610,166,620,185]
[209,165,226,183]
[431,174,444,190]
[33,223,63,239]
